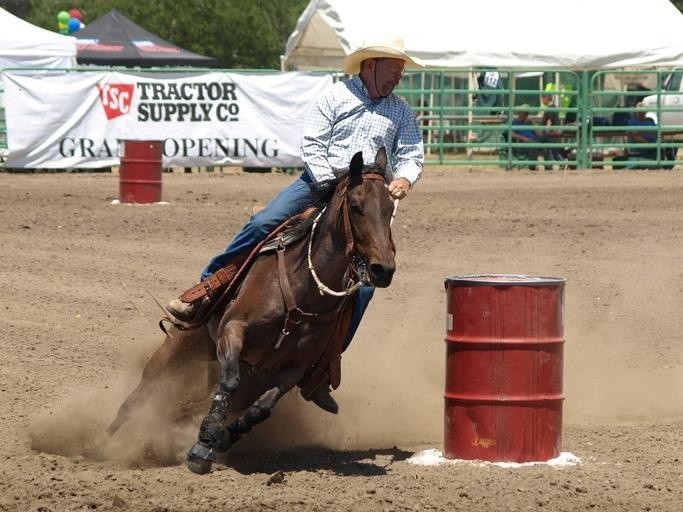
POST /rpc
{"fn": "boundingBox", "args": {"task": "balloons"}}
[57,6,85,34]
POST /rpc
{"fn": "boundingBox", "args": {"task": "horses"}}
[99,145,396,475]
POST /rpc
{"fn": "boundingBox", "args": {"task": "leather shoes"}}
[296,367,339,414]
[165,295,201,322]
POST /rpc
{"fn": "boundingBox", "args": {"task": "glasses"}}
[381,60,406,75]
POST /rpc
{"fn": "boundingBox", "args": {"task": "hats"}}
[343,38,427,76]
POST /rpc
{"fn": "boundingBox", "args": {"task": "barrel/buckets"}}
[441,272,567,464]
[116,137,164,204]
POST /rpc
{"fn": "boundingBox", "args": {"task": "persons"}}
[474,67,656,170]
[167,30,425,354]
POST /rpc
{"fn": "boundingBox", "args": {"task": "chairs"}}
[495,111,682,171]
